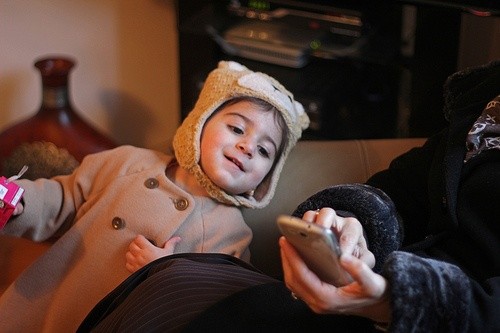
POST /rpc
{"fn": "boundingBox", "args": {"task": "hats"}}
[174,60,310,209]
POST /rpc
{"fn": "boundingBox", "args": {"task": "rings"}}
[290,291,297,299]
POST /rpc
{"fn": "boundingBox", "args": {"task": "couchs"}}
[238,137,430,272]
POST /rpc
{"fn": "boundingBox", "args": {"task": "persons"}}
[75,57,500,333]
[0,60,311,333]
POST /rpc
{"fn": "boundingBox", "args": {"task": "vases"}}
[0,55,120,181]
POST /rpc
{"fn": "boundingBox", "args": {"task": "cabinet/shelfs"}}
[173,0,464,139]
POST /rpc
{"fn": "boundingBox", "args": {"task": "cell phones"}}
[276,215,355,287]
